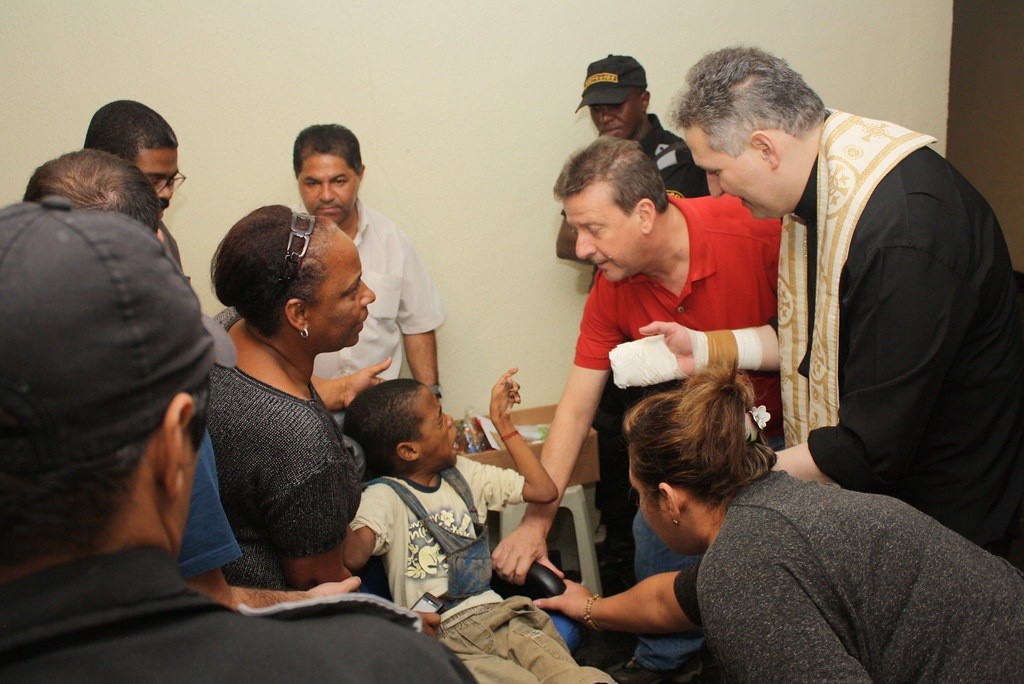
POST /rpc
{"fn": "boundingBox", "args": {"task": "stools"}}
[490,486,604,604]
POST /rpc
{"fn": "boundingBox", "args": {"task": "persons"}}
[0,100,479,684]
[609,47,1024,574]
[205,205,392,591]
[557,53,709,593]
[293,125,445,422]
[490,136,783,584]
[532,356,1024,684]
[342,370,617,684]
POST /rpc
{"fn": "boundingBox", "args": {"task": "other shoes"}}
[594,537,634,573]
[605,655,703,684]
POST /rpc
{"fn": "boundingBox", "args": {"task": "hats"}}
[575,55,648,114]
[0,194,236,462]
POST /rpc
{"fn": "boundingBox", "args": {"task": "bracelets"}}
[501,431,518,441]
[429,384,442,398]
[584,594,602,632]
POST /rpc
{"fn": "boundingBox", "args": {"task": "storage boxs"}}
[452,401,601,488]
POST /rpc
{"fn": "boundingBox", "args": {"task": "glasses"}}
[148,172,186,193]
